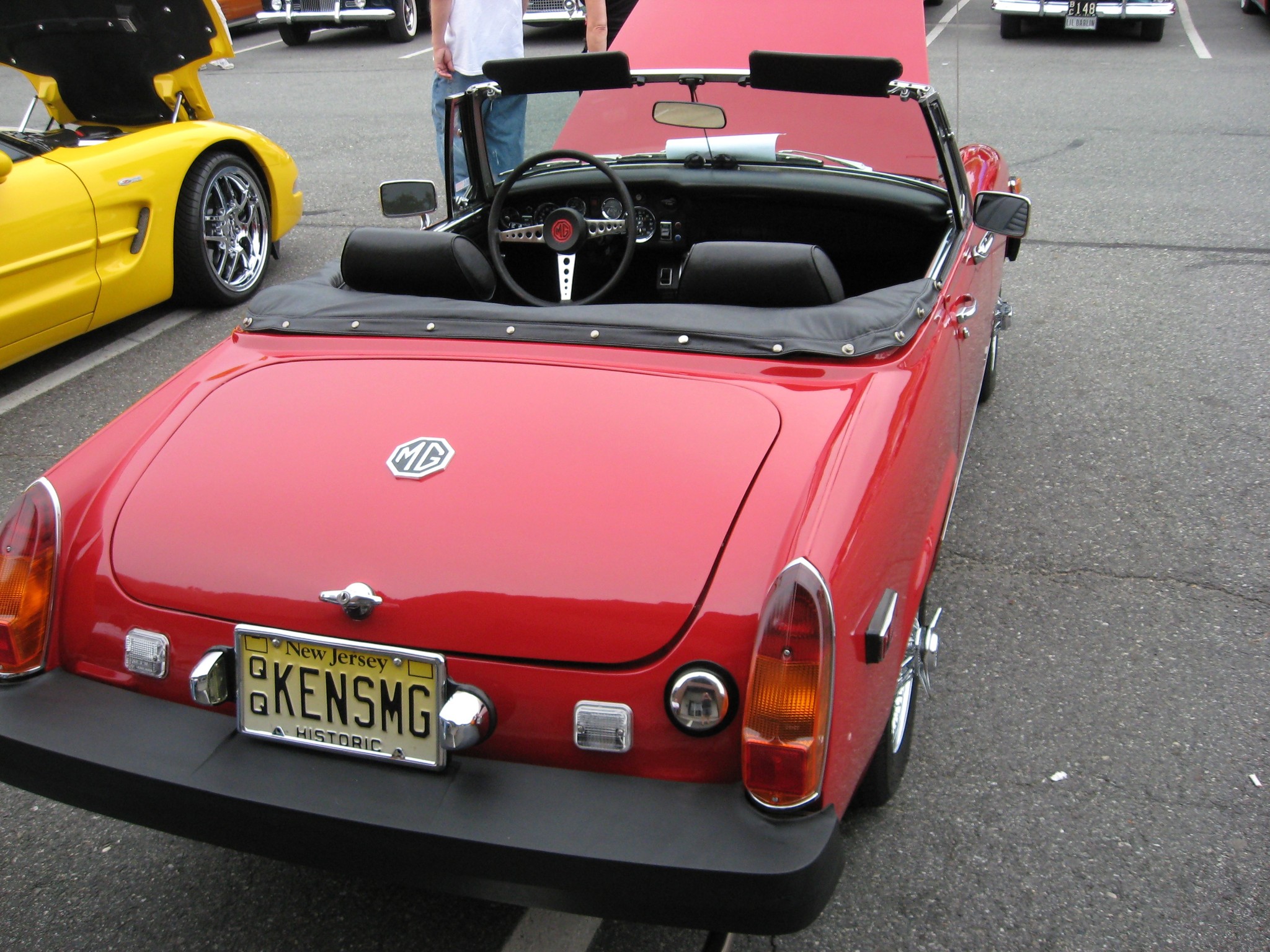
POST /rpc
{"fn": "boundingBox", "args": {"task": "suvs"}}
[521,0,587,25]
[990,1,1175,41]
[256,0,431,48]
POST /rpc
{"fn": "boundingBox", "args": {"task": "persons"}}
[431,0,528,209]
[577,1,641,99]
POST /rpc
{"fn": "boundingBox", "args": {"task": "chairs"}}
[673,241,846,307]
[341,228,500,304]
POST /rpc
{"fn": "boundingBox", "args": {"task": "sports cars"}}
[0,49,1037,932]
[0,0,305,380]
